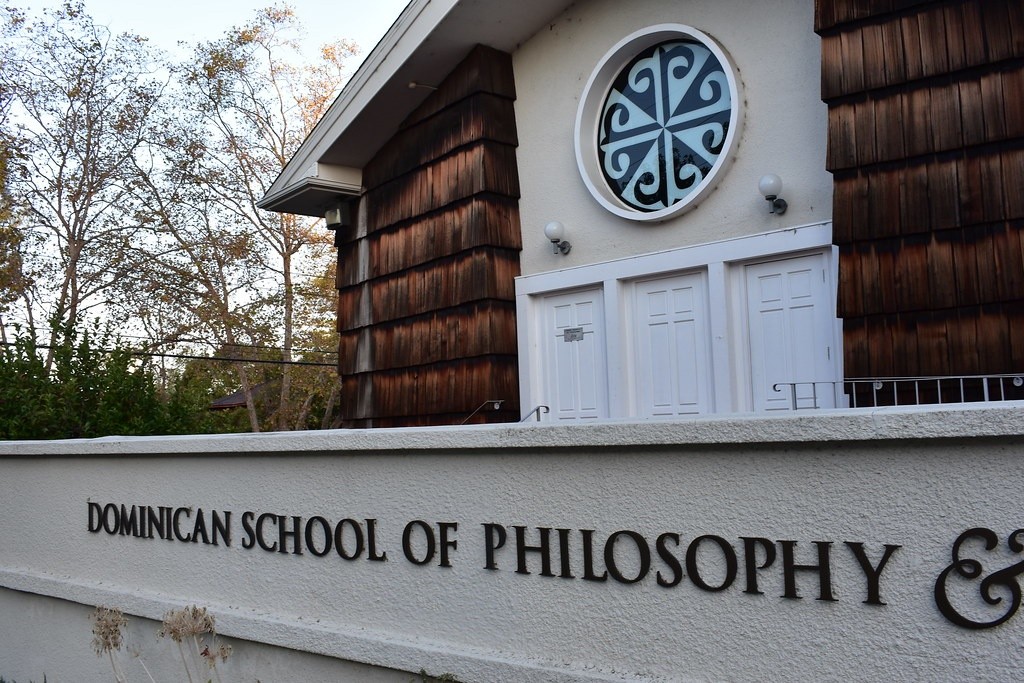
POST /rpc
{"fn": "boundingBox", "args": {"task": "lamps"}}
[544,220,572,257]
[758,173,787,215]
